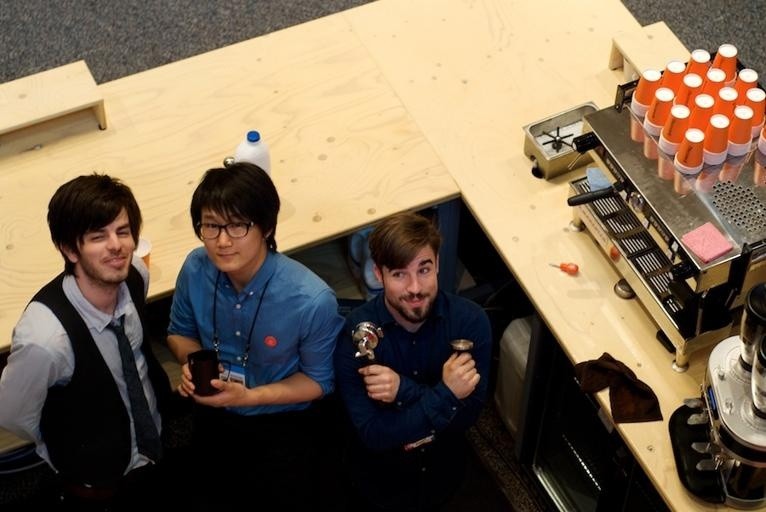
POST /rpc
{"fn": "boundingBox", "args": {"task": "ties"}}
[106,314,165,464]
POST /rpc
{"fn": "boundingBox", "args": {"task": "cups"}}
[187,349,232,396]
[631,44,766,175]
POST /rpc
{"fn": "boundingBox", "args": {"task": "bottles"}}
[234,130,271,180]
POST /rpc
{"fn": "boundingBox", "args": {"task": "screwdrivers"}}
[549,263,578,273]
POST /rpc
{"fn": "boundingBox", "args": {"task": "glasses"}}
[195,220,255,240]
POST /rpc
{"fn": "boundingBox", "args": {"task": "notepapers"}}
[680,221,734,264]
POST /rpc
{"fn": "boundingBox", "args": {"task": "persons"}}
[165,159,346,512]
[334,212,494,512]
[0,171,175,510]
